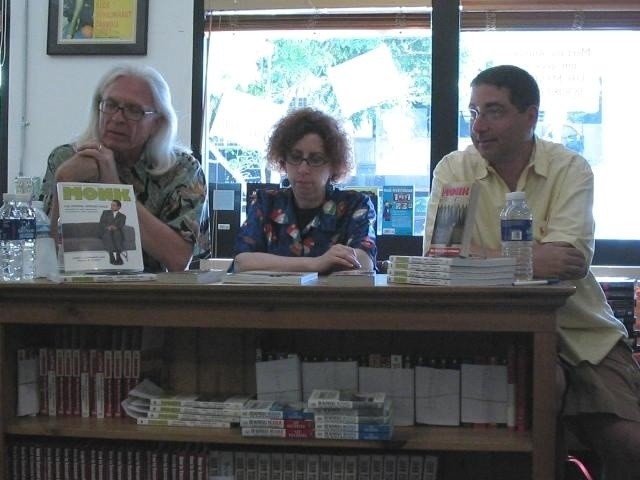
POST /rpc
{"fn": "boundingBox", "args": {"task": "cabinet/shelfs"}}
[1,274,576,480]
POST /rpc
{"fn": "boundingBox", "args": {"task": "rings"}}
[98,144,102,152]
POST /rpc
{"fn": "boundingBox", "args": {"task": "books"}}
[596,277,640,352]
[137,393,257,428]
[209,445,446,480]
[327,270,376,287]
[303,353,356,362]
[461,354,527,432]
[314,401,395,440]
[48,273,156,284]
[348,179,516,287]
[417,355,457,370]
[16,344,168,418]
[359,351,414,368]
[240,400,315,438]
[256,347,297,362]
[308,389,385,409]
[223,272,318,284]
[56,182,144,273]
[1,436,209,479]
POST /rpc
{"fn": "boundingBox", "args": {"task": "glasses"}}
[283,155,333,168]
[97,97,156,121]
[463,107,528,124]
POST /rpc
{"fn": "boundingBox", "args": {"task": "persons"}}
[423,65,640,479]
[235,109,377,274]
[98,200,126,264]
[39,62,206,272]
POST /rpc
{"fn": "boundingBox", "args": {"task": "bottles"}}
[0,193,64,281]
[499,193,534,281]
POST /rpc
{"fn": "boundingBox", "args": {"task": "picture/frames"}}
[46,0,148,56]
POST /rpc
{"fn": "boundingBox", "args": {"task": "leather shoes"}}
[110,257,123,265]
